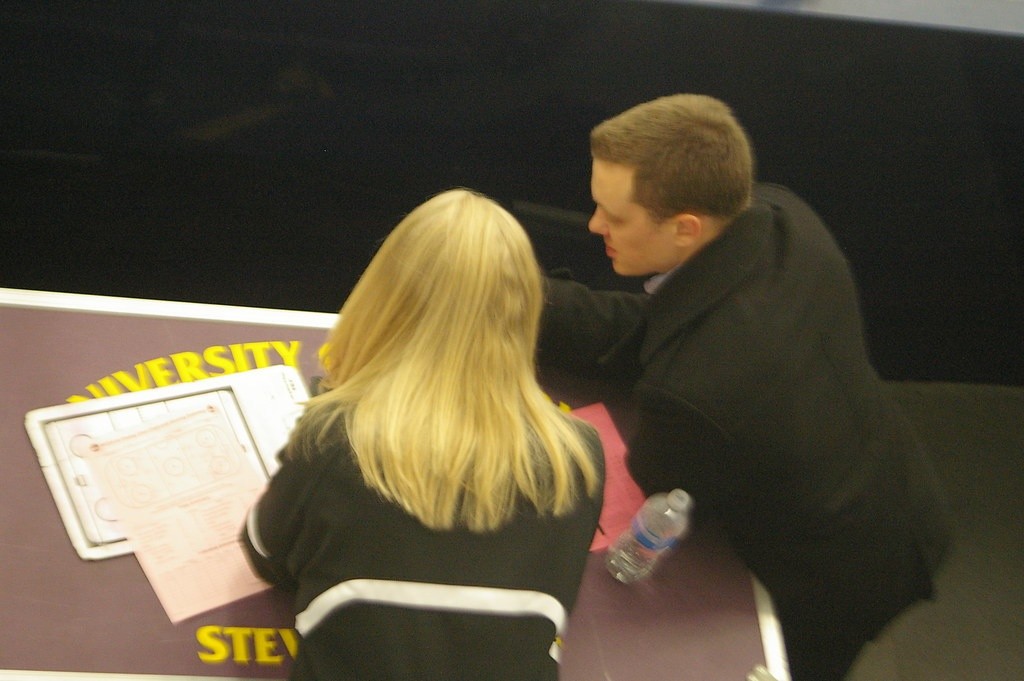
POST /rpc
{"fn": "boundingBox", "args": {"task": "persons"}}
[534,95,950,681]
[240,190,607,681]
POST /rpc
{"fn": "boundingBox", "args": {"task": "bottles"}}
[606,489,691,585]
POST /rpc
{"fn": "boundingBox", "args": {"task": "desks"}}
[0,287,791,681]
